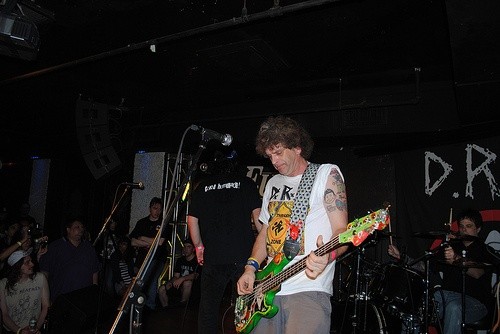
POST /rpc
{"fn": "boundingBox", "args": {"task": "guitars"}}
[234,202,390,334]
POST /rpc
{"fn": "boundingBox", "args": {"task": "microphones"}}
[122,182,143,189]
[190,124,233,147]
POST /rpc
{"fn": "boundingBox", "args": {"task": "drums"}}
[331,298,388,334]
[399,312,428,334]
[340,259,381,300]
[420,299,440,325]
[371,263,425,315]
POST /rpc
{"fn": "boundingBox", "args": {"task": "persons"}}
[236,116,350,334]
[388,209,495,334]
[0,197,202,334]
[188,141,263,334]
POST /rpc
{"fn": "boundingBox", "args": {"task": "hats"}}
[8,247,33,267]
[184,239,193,244]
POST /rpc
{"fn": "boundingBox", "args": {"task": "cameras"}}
[34,235,48,248]
[27,227,36,236]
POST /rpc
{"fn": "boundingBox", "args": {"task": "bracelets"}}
[245,257,260,272]
[16,242,21,246]
[331,251,336,262]
[17,328,22,334]
[196,246,204,250]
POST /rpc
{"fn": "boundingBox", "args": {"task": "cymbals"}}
[408,232,482,242]
[372,229,401,240]
[436,259,495,268]
